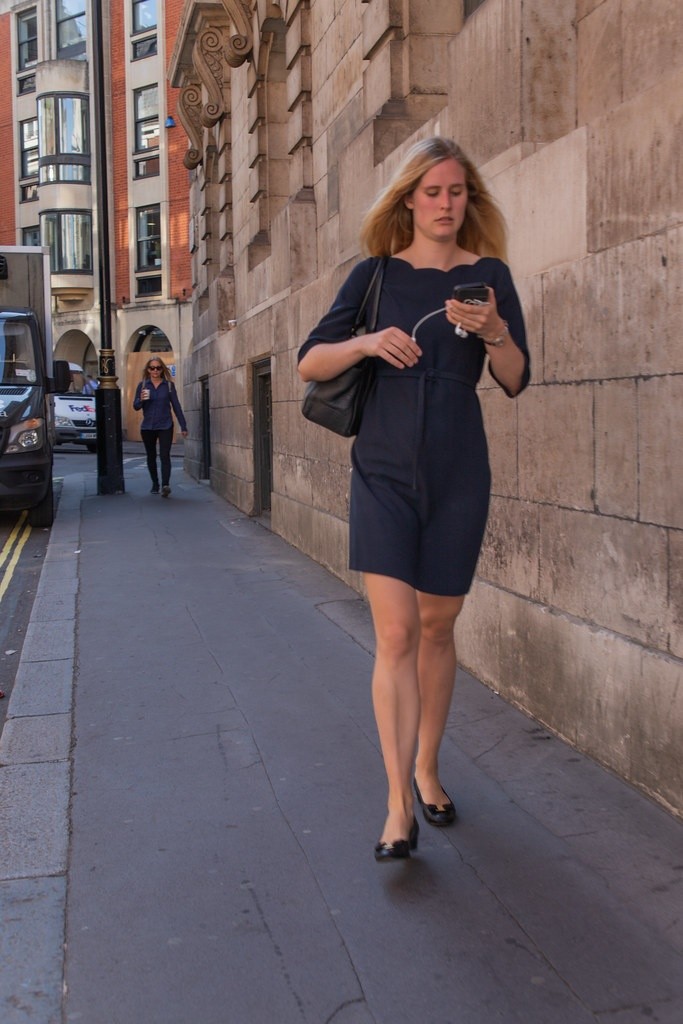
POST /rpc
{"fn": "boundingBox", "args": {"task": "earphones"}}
[455,322,463,335]
[461,330,467,338]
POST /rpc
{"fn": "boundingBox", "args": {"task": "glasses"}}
[147,366,162,370]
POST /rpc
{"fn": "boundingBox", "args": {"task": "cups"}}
[142,390,150,399]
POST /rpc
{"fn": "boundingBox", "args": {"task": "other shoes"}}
[150,482,159,493]
[162,485,171,497]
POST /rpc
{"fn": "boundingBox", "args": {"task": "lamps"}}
[164,116,175,127]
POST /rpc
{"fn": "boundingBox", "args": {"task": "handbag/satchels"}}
[301,254,387,437]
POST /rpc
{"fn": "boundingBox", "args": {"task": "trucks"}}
[0,246,73,528]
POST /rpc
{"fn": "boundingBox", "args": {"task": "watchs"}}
[479,321,509,346]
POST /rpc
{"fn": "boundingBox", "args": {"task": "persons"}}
[79,373,97,395]
[296,132,535,859]
[117,355,187,497]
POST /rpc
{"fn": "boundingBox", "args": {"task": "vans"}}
[51,359,97,454]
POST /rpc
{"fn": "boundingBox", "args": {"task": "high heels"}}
[373,812,419,861]
[413,774,456,827]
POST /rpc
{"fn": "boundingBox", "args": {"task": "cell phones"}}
[453,282,488,306]
